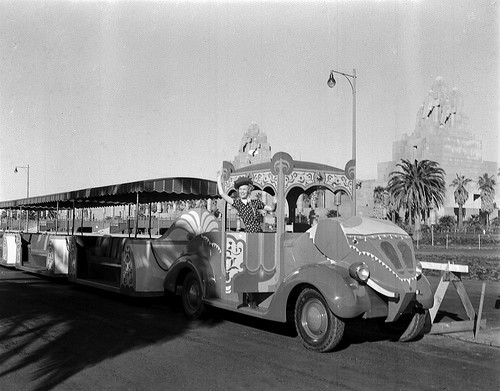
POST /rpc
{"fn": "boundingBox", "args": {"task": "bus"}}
[0,152,434,354]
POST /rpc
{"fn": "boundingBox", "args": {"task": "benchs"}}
[86,238,127,267]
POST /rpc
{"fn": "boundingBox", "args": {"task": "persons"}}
[217,170,277,234]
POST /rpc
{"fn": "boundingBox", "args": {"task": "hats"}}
[234,177,253,189]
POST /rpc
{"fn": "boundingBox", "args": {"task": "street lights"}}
[14,164,29,197]
[328,68,356,160]
